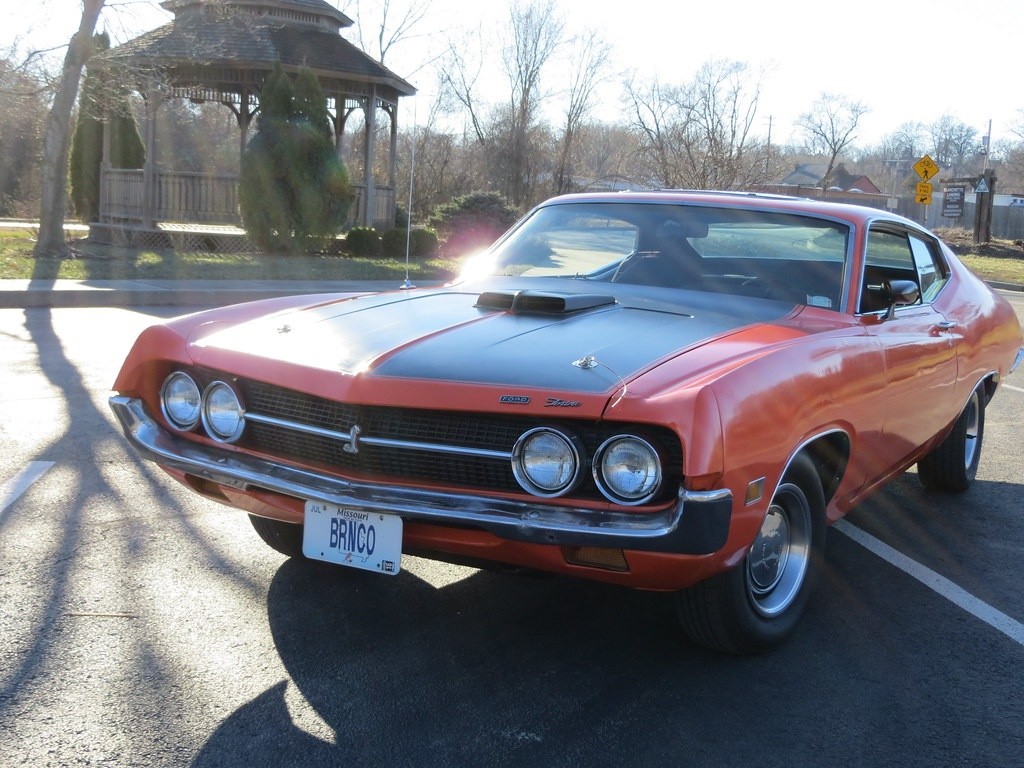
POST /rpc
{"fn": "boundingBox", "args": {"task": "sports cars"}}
[106,189,1022,655]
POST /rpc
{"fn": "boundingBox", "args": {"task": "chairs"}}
[770,261,825,295]
[615,257,675,288]
